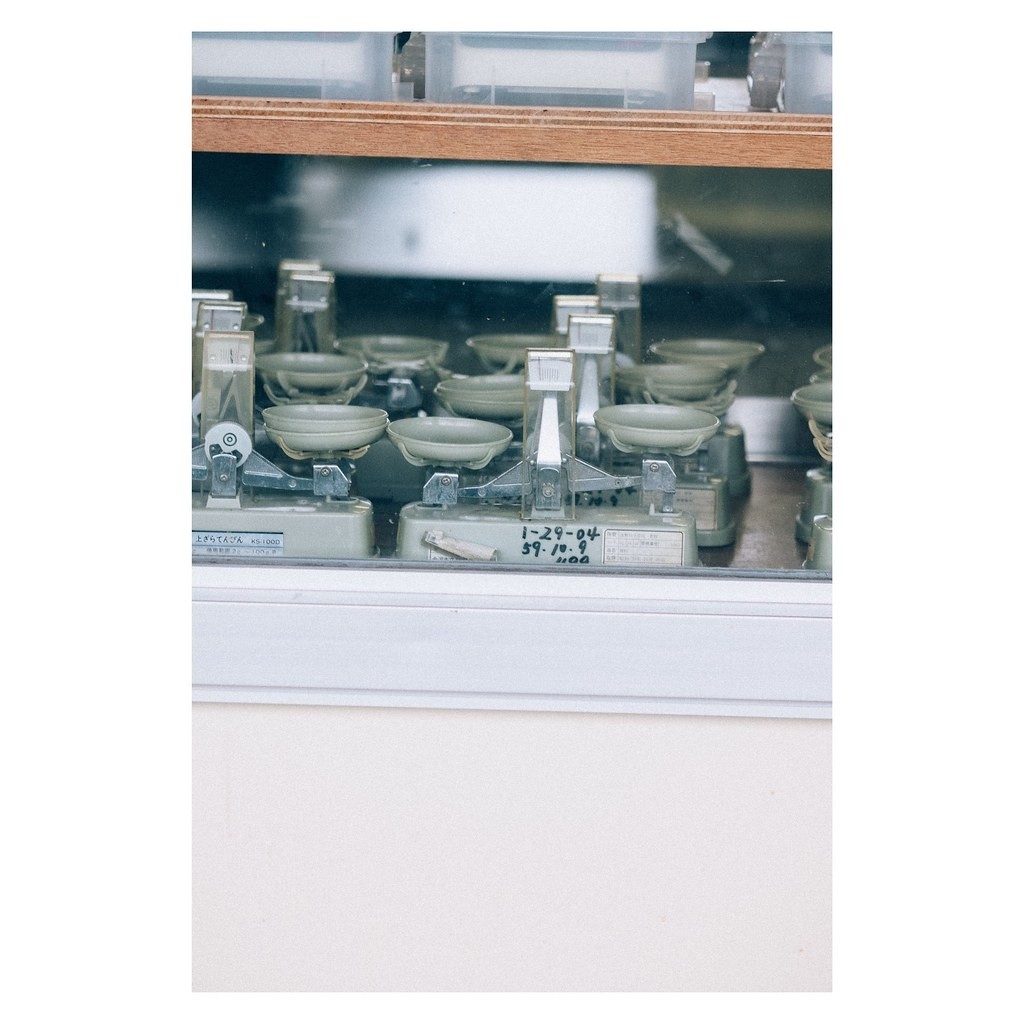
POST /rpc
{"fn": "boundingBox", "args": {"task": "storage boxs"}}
[421,32,716,112]
[762,32,832,115]
[192,31,401,104]
